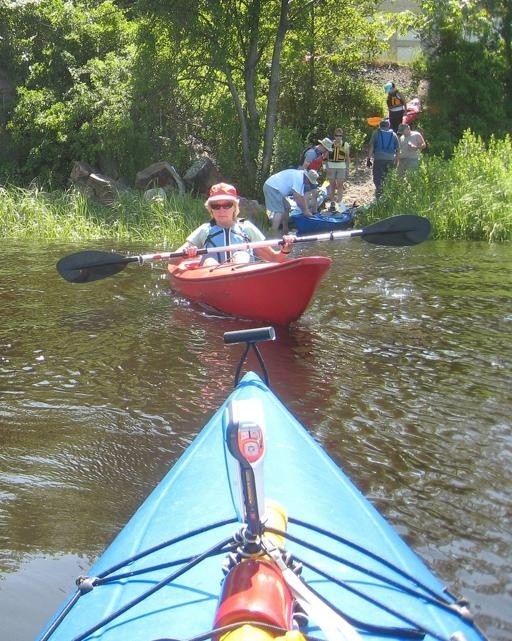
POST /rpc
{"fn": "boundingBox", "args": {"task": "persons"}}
[397,124,427,176]
[324,128,350,212]
[384,80,407,135]
[263,169,319,235]
[402,97,424,125]
[366,119,400,201]
[297,137,333,216]
[166,182,299,269]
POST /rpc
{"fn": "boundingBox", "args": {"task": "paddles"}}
[56,213,431,284]
[367,101,444,126]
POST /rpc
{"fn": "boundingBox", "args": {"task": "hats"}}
[304,168,318,185]
[207,182,238,204]
[397,123,409,133]
[318,137,334,153]
[383,80,393,93]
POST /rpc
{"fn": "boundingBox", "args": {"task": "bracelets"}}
[346,167,349,170]
[280,249,292,255]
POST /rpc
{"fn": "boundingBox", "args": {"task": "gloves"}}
[366,158,373,168]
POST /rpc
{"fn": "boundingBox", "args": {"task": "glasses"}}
[209,202,234,211]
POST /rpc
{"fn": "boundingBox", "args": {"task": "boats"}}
[383,96,423,129]
[266,184,353,237]
[166,242,332,327]
